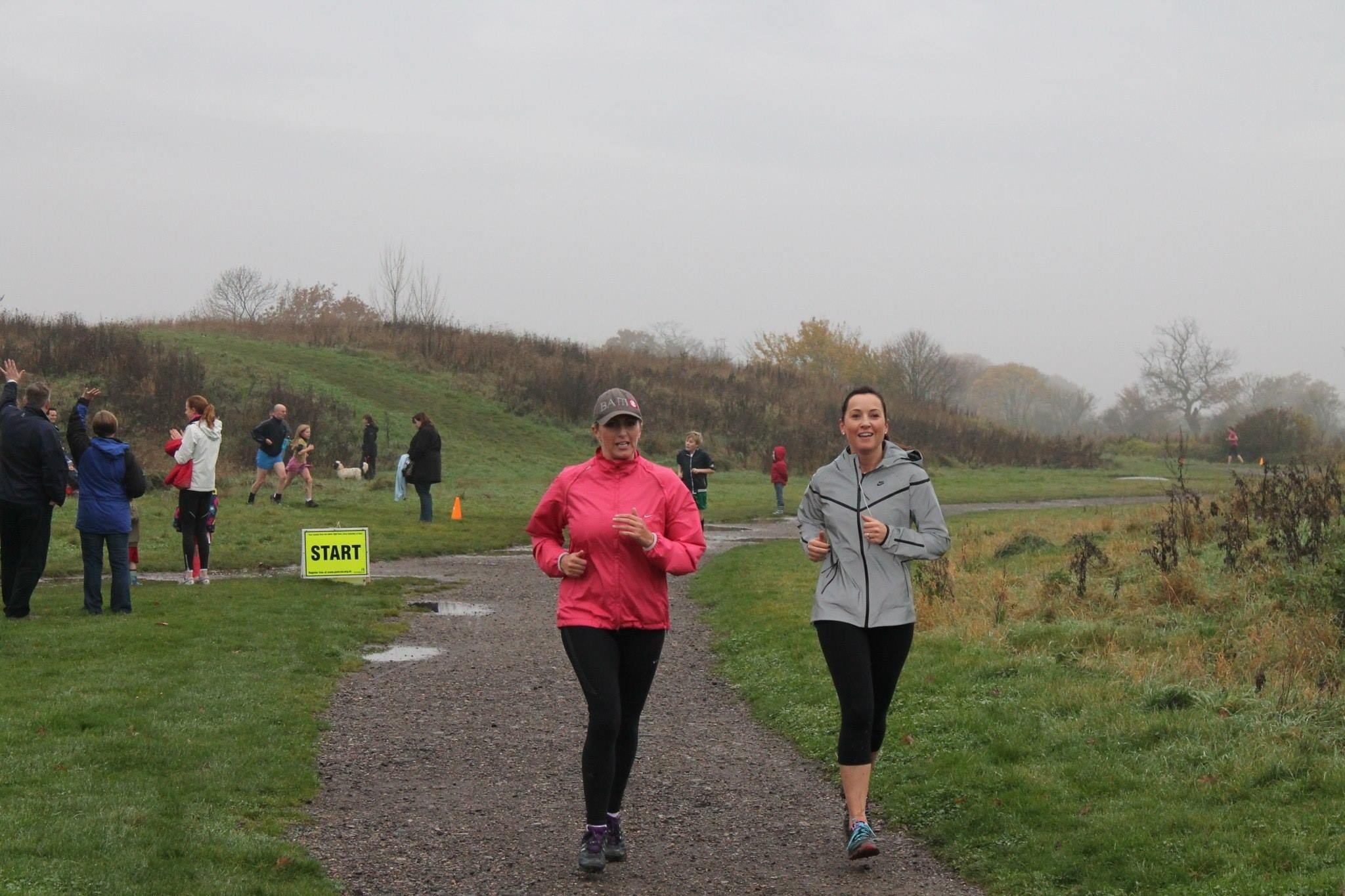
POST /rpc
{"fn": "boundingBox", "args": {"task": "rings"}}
[867,533,870,538]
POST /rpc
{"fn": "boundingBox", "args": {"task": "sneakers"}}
[243,495,318,508]
[579,824,607,869]
[843,796,880,859]
[602,812,627,861]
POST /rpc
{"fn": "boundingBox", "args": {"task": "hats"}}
[591,388,643,426]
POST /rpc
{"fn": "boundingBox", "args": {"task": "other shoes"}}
[130,573,212,586]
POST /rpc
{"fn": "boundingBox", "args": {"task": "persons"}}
[770,446,789,515]
[245,404,288,507]
[65,456,80,493]
[46,408,65,452]
[795,384,951,863]
[676,430,716,533]
[67,386,148,617]
[127,497,144,586]
[172,488,219,583]
[265,405,292,489]
[0,357,67,620]
[169,394,223,586]
[403,412,442,523]
[1224,426,1246,467]
[359,414,379,480]
[269,425,319,508]
[523,389,707,876]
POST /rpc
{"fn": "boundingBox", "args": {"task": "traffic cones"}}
[448,496,463,523]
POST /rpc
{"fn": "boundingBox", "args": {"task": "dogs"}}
[332,460,369,481]
